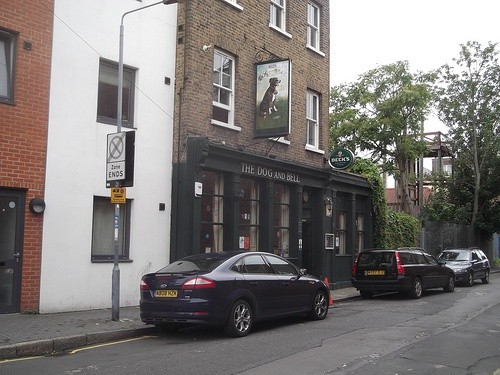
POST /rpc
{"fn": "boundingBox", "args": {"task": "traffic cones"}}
[324,276,334,305]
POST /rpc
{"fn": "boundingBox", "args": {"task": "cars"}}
[140,249,330,338]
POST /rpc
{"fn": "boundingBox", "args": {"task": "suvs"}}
[437,246,491,288]
[350,246,457,299]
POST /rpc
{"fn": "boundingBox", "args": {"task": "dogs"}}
[259,77,282,119]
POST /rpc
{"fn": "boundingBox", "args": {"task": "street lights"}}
[111,0,181,322]
